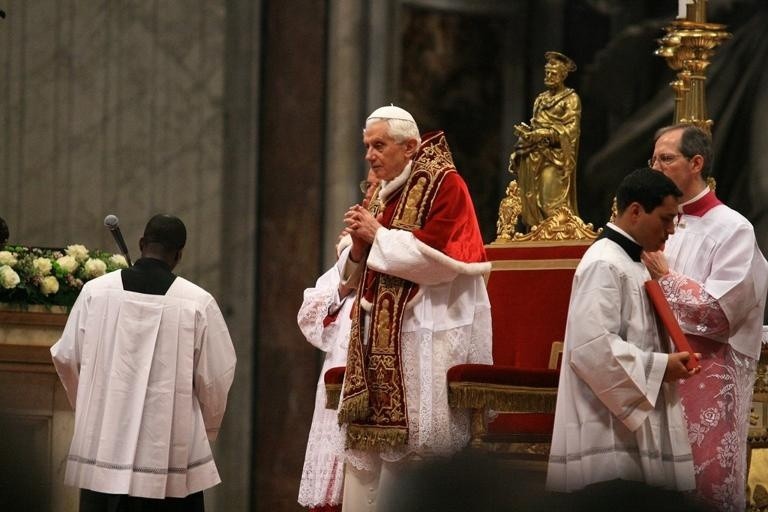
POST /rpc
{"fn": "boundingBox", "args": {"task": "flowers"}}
[0,243,137,313]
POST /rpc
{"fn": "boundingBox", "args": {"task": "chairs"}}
[324,241,594,474]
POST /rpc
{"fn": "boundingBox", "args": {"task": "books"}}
[642,276,703,374]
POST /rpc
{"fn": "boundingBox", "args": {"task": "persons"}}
[46,208,242,508]
[293,166,380,508]
[532,161,704,508]
[504,49,590,222]
[634,121,765,510]
[335,103,495,510]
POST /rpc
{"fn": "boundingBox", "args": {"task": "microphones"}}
[103,215,133,268]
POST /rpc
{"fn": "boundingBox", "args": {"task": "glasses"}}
[645,152,686,169]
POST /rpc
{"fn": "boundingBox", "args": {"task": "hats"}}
[365,101,417,127]
